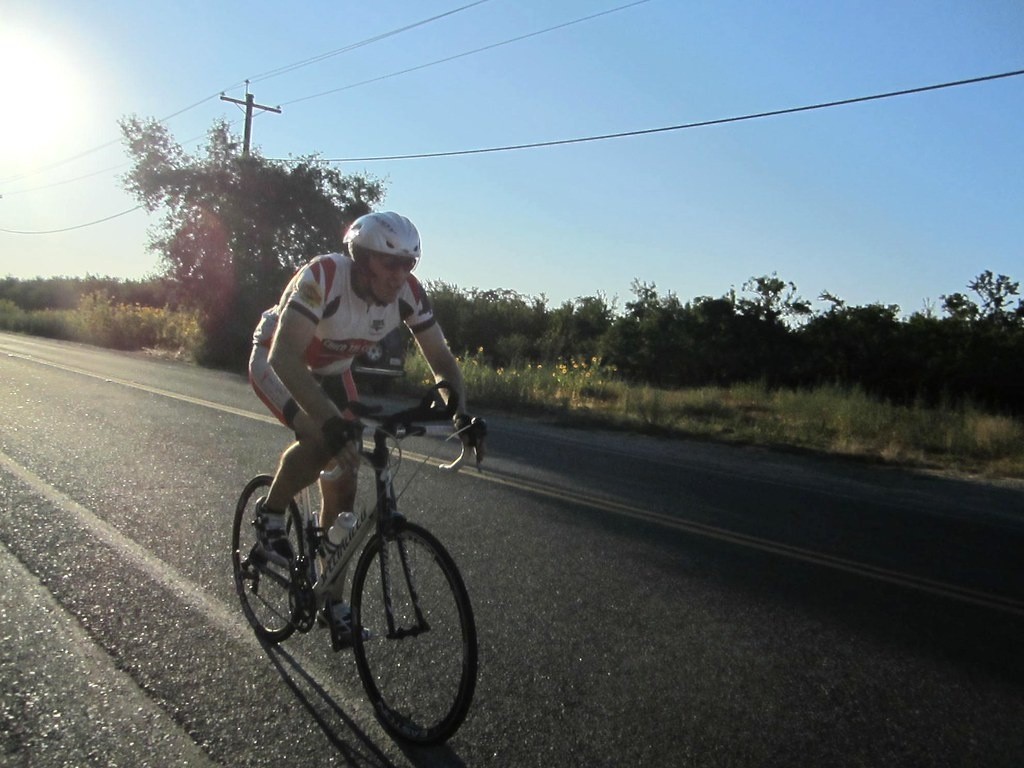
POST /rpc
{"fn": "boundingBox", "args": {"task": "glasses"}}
[367,254,416,272]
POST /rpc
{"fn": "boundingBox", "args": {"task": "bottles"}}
[326,512,357,547]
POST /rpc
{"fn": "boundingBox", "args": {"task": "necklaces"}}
[247,212,487,640]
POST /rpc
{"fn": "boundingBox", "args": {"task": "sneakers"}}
[251,496,297,567]
[316,600,370,643]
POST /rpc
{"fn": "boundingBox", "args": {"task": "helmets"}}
[341,211,420,257]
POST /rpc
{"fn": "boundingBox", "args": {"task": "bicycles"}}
[231,398,496,743]
